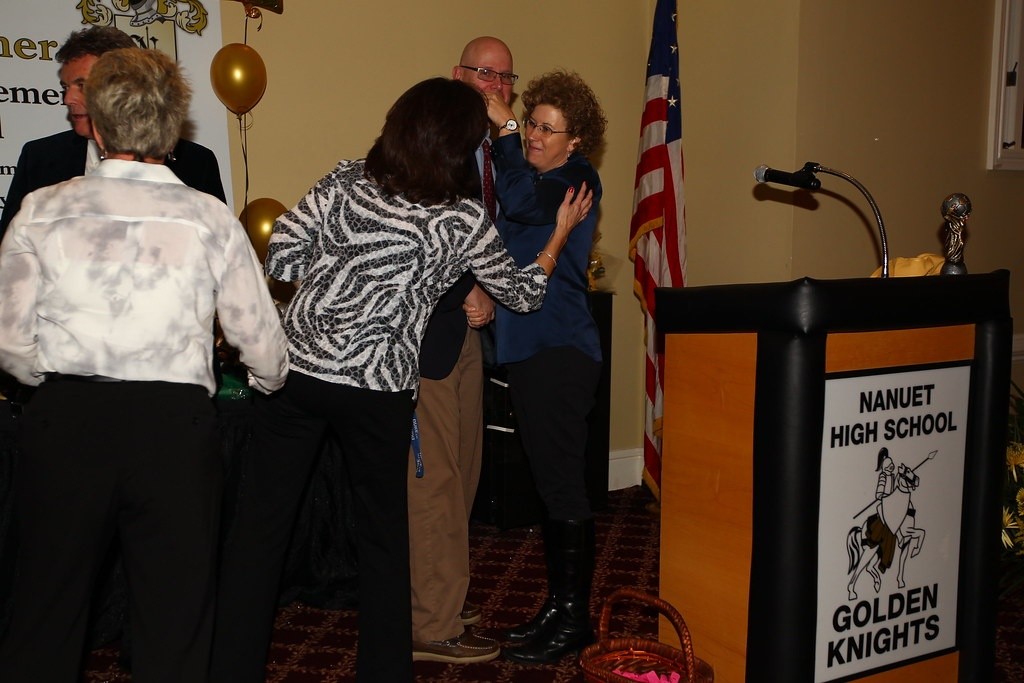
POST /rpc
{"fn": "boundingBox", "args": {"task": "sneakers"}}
[412,599,500,664]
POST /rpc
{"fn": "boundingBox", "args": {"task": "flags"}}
[627,0,685,506]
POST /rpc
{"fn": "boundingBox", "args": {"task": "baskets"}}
[578,588,714,683]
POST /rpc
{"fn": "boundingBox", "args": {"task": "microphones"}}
[753,164,821,190]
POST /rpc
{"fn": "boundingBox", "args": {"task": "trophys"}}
[940,193,972,275]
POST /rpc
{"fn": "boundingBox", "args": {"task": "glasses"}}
[458,63,520,86]
[524,117,573,138]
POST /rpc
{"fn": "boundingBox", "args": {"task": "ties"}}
[482,140,496,224]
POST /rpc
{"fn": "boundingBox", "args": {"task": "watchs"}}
[498,118,521,131]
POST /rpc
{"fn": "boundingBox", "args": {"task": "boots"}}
[503,514,599,662]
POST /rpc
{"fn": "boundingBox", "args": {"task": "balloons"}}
[239,197,288,265]
[210,43,268,118]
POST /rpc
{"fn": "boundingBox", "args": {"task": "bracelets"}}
[537,252,557,266]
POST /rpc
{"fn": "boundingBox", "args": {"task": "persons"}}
[0,26,289,683]
[209,78,593,683]
[408,35,606,664]
